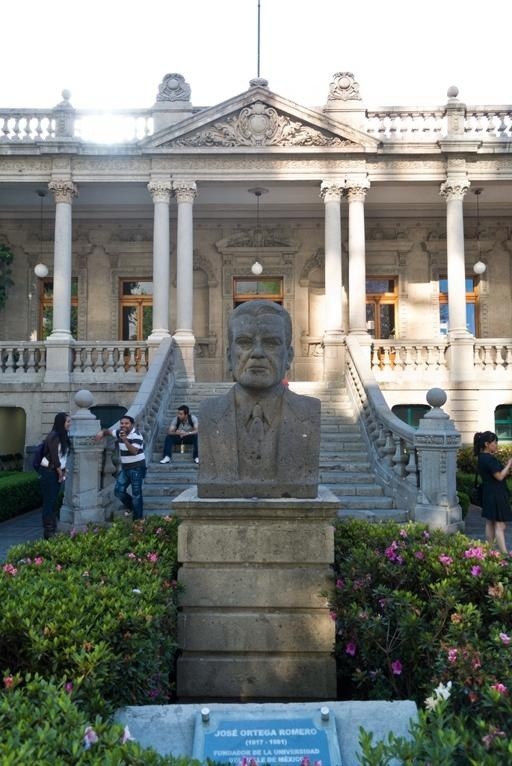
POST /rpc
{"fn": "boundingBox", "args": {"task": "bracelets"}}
[188,431,192,436]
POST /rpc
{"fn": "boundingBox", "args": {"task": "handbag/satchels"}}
[32,439,49,474]
[470,481,483,508]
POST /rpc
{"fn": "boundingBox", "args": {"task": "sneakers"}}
[125,508,134,516]
[194,457,199,463]
[160,455,171,463]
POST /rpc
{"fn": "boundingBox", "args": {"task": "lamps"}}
[32,188,50,279]
[468,187,487,274]
[247,188,269,276]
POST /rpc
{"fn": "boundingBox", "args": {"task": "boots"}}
[42,511,56,539]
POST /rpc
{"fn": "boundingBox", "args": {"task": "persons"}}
[198,299,321,498]
[96,416,147,521]
[160,404,198,464]
[474,431,512,554]
[35,412,72,540]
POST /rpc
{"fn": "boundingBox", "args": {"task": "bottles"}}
[180,439,185,454]
